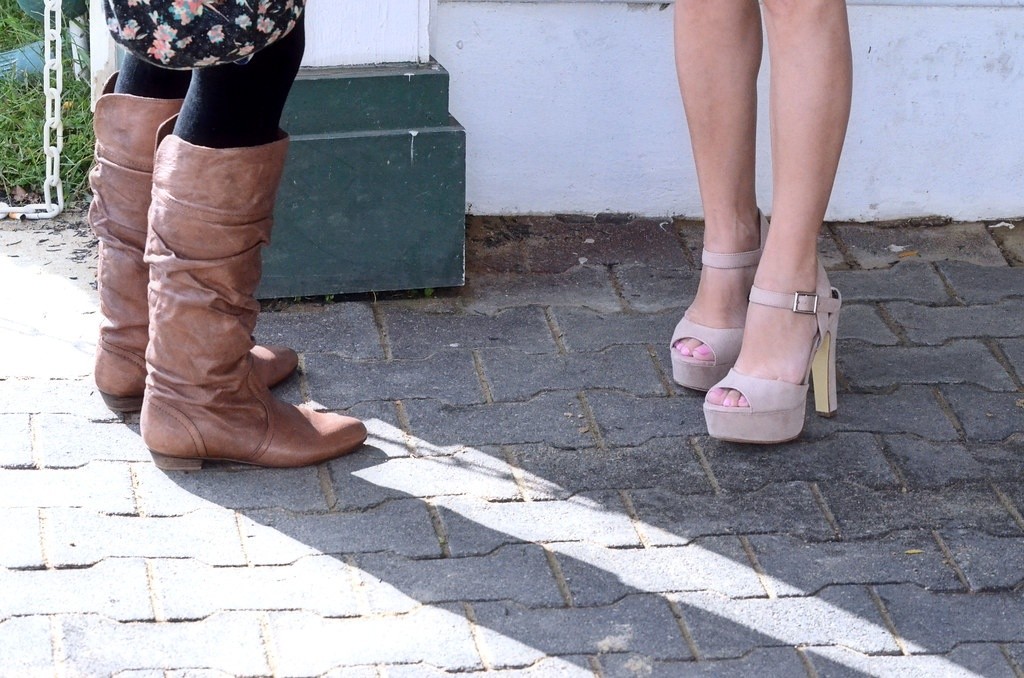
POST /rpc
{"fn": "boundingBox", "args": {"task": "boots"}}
[89,72,301,412]
[140,114,368,469]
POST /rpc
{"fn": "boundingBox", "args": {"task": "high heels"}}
[701,256,842,444]
[671,208,769,391]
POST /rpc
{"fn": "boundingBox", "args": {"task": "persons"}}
[671,1,852,444]
[92,0,366,470]
